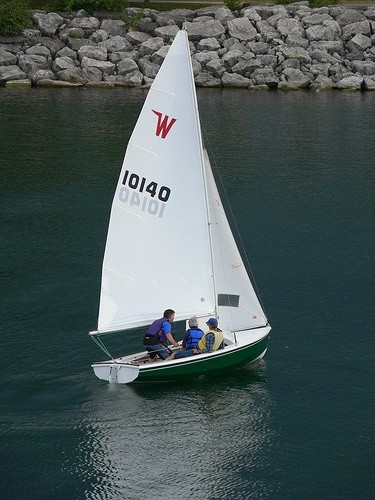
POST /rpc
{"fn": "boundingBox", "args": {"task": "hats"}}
[189,318,198,326]
[206,318,217,325]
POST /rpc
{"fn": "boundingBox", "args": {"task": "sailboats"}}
[88,25,272,383]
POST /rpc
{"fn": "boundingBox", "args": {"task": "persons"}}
[142,309,179,362]
[175,318,224,359]
[182,318,204,350]
